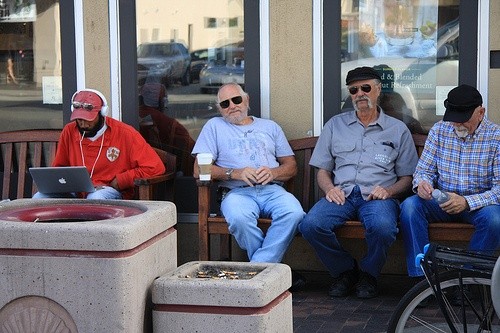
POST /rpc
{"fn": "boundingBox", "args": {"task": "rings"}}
[267,174,270,177]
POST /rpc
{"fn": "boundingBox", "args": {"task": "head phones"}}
[70,88,110,117]
[138,82,168,110]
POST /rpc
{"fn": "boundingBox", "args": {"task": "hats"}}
[141,83,166,107]
[442,85,483,123]
[345,66,381,85]
[70,91,102,121]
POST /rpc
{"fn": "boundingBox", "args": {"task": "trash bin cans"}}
[150,260,293,333]
[0,197,178,333]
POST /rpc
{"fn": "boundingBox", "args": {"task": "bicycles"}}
[387,241,500,333]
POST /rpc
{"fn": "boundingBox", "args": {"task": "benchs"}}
[196,135,479,262]
[0,129,177,203]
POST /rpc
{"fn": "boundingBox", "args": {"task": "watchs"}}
[225,168,234,181]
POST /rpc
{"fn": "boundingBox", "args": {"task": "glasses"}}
[218,95,243,110]
[72,102,101,112]
[444,98,482,110]
[349,84,372,95]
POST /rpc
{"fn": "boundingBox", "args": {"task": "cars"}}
[138,42,193,87]
[190,41,244,95]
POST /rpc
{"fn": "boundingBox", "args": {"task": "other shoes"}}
[328,258,358,297]
[417,285,470,307]
[355,272,379,298]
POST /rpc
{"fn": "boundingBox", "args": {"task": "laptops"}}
[29,166,98,193]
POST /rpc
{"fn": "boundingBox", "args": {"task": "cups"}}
[196,153,214,181]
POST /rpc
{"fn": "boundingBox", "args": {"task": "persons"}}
[5,58,17,84]
[138,83,196,177]
[33,89,166,201]
[299,66,419,299]
[400,84,500,306]
[191,82,308,291]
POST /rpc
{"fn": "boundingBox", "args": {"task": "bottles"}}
[431,189,451,204]
